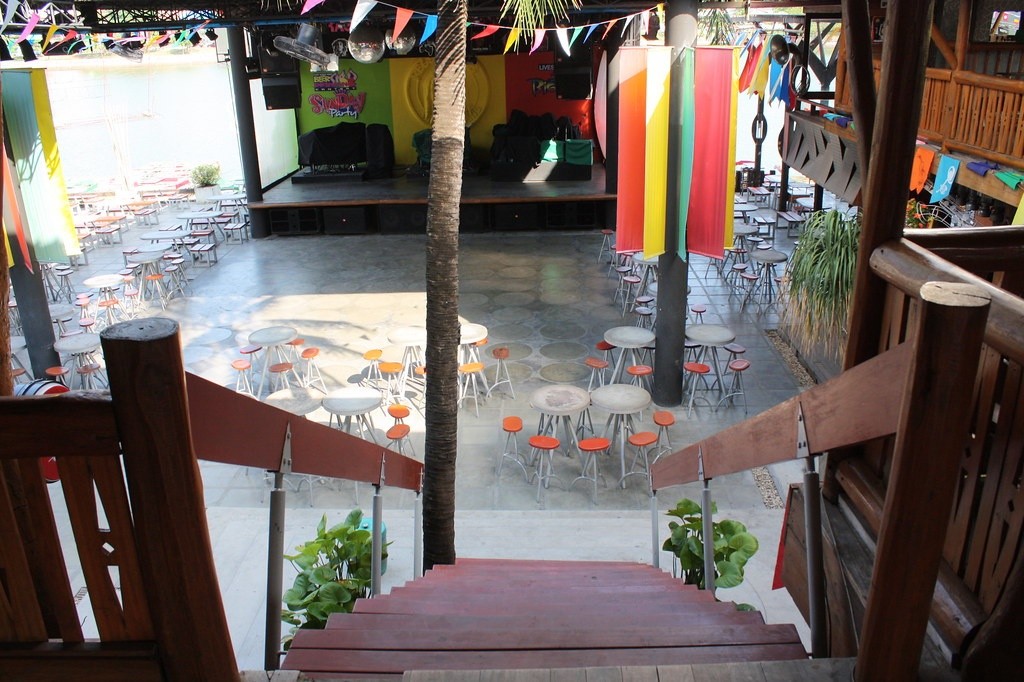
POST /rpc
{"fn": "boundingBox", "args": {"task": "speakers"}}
[554,39,594,101]
[255,45,302,111]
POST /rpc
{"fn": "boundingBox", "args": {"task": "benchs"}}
[734,195,776,241]
[776,211,805,238]
[69,192,248,271]
[747,187,771,210]
[763,178,781,192]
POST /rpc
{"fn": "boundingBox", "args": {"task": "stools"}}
[231,338,516,457]
[498,230,792,503]
[7,263,184,389]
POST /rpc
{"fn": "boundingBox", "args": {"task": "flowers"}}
[905,198,928,229]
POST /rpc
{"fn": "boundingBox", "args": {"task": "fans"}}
[103,32,143,60]
[273,23,330,67]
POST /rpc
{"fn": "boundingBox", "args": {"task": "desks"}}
[143,192,175,197]
[54,334,109,391]
[765,175,795,210]
[48,303,72,333]
[389,327,427,400]
[10,336,33,384]
[602,326,656,395]
[734,203,758,223]
[95,216,130,231]
[627,254,658,312]
[788,182,810,210]
[307,388,383,510]
[124,200,163,213]
[127,252,165,312]
[587,384,652,488]
[644,283,692,331]
[246,388,323,504]
[530,384,590,489]
[83,274,131,330]
[685,325,736,418]
[137,243,191,290]
[176,212,224,246]
[140,230,191,256]
[458,324,492,419]
[249,326,304,400]
[721,224,758,273]
[796,198,832,220]
[207,194,248,220]
[745,250,788,314]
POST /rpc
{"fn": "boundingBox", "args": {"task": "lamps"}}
[0,28,218,62]
[642,8,660,40]
[253,21,417,63]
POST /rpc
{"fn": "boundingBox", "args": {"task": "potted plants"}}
[191,163,222,202]
